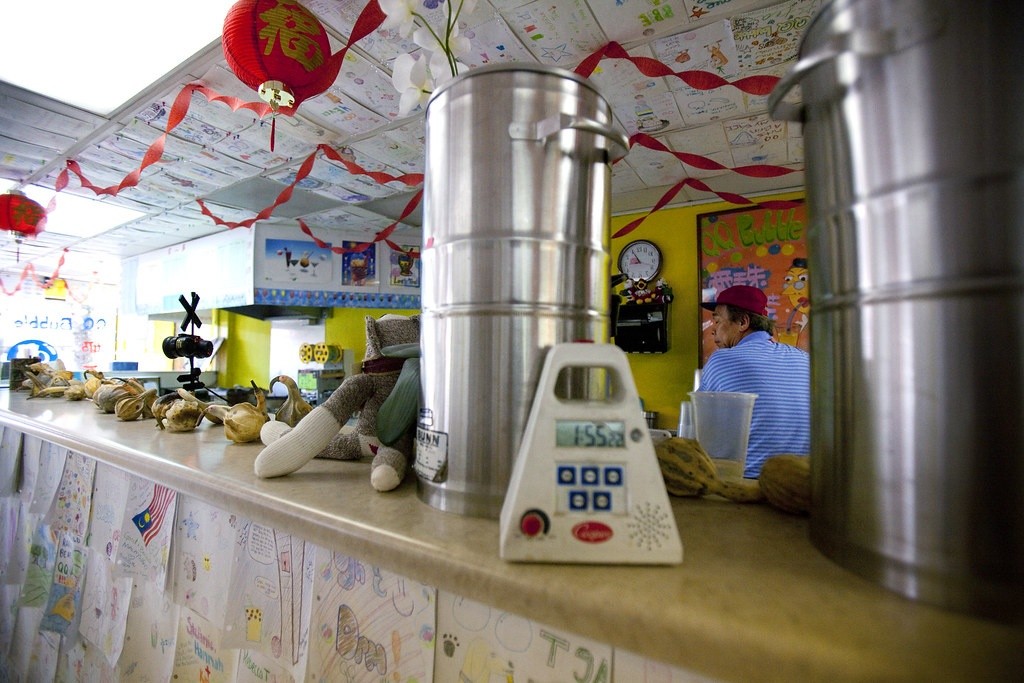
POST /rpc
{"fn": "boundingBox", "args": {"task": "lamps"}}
[161,292,213,400]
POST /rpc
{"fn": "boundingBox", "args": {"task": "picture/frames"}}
[697,198,809,369]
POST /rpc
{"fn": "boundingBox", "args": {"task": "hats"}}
[699,285,768,315]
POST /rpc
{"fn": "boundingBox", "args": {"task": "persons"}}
[695,285,811,480]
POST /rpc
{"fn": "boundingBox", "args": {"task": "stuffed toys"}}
[254,312,421,493]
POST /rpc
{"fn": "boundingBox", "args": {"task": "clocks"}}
[617,239,663,283]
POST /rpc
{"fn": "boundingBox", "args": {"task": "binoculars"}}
[162,333,213,358]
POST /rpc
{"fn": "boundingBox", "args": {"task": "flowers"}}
[378,0,478,119]
